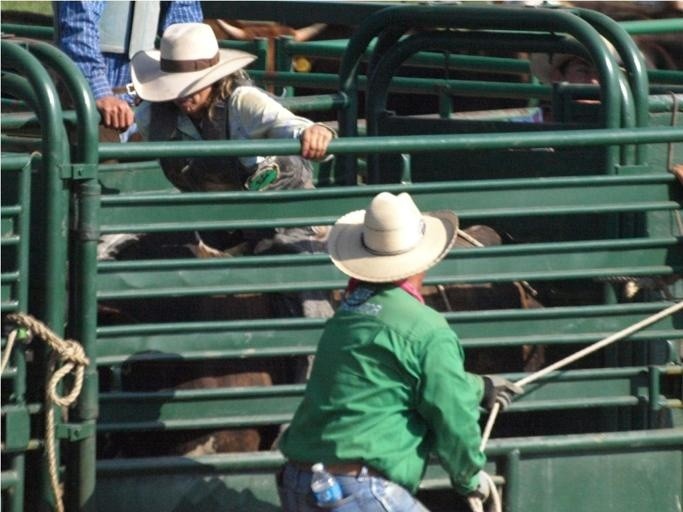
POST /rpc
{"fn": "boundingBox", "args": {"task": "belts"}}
[288,461,379,476]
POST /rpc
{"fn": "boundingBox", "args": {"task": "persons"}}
[99,23,343,258]
[198,1,351,124]
[272,191,529,511]
[50,0,207,164]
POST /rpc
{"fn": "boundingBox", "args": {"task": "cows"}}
[96,226,551,512]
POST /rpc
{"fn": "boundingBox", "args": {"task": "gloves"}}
[484,374,524,413]
[466,469,506,512]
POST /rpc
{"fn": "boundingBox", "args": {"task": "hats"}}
[327,191,459,283]
[129,22,259,103]
